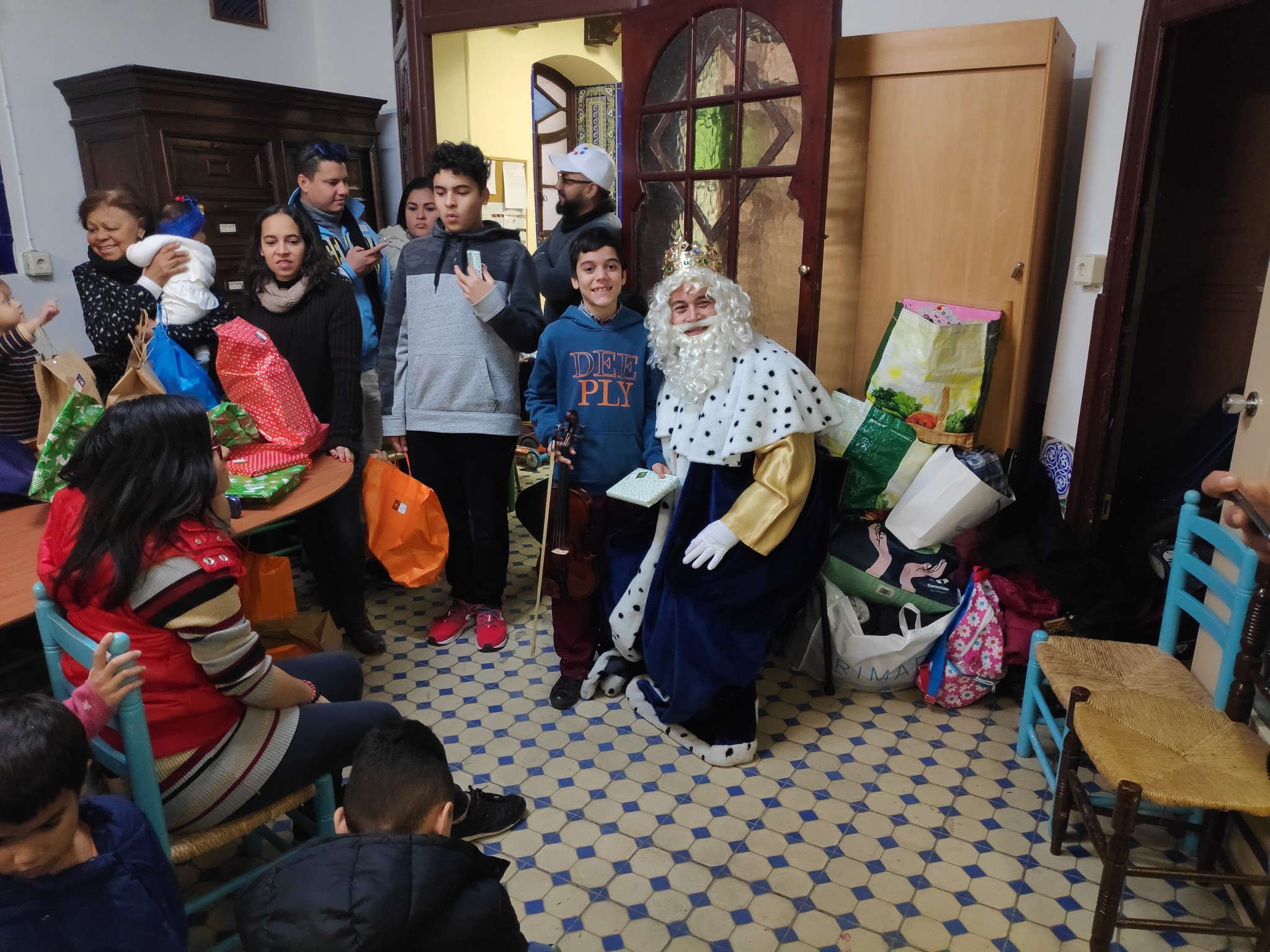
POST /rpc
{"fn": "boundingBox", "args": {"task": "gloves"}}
[681,518,739,571]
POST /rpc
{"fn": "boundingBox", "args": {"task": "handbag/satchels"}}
[31,324,104,450]
[148,303,220,417]
[358,447,450,591]
[105,307,168,407]
[788,297,1063,713]
[230,536,297,618]
[249,611,341,653]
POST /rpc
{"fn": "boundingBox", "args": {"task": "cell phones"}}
[380,235,391,243]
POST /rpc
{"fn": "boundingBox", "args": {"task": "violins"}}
[526,409,600,604]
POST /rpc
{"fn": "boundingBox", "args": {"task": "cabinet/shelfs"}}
[51,64,388,310]
[719,15,1077,459]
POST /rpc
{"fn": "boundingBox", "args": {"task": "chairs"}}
[1048,567,1270,952]
[1014,490,1260,853]
[33,582,335,916]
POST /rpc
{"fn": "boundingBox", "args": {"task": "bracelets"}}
[18,323,37,344]
[301,677,317,703]
[310,686,321,703]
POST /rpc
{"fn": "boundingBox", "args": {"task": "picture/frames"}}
[209,0,268,29]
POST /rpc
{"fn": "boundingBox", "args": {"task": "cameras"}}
[467,250,484,281]
[223,494,242,519]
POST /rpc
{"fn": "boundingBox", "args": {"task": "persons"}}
[0,139,835,844]
[0,695,190,952]
[234,720,531,952]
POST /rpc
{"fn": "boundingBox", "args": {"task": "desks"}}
[0,454,354,677]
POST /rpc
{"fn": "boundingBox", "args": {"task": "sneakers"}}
[452,784,529,843]
[425,596,477,645]
[471,603,507,653]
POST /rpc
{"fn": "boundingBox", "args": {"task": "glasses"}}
[212,435,224,460]
[557,172,593,189]
[299,141,350,165]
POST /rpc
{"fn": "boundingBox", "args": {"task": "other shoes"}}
[342,607,387,654]
[550,676,581,710]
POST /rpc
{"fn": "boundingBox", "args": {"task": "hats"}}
[549,143,617,191]
